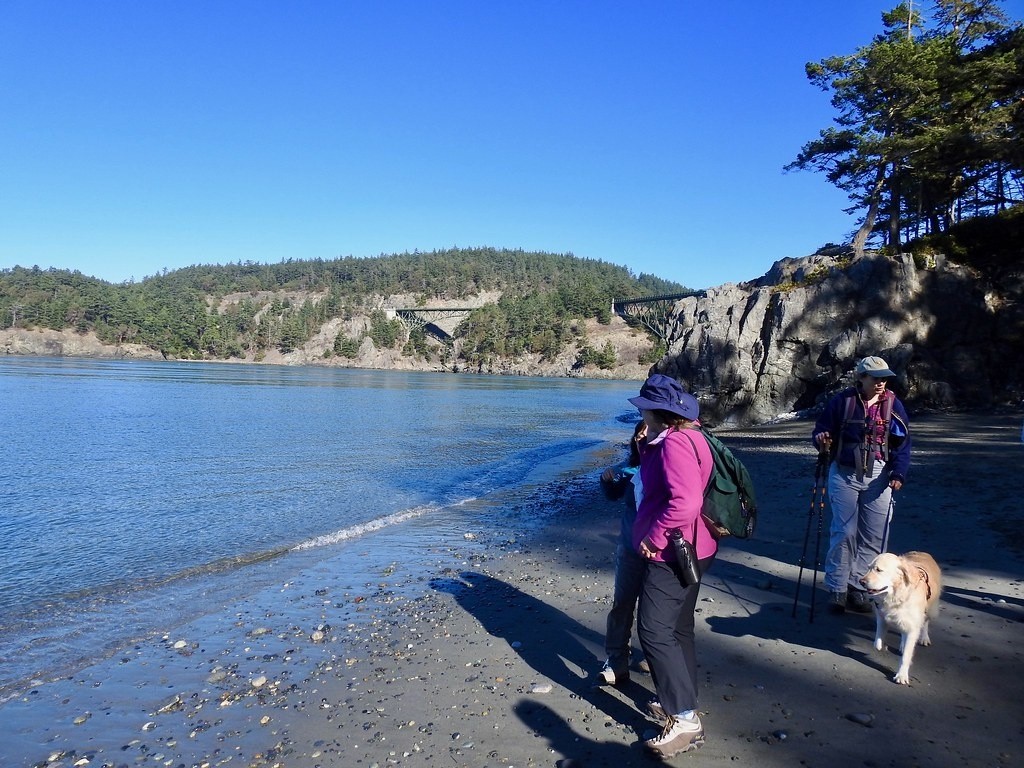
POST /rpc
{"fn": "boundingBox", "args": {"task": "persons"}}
[584,419,650,686]
[812,356,910,613]
[628,373,719,759]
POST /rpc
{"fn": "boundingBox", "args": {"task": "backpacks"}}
[689,419,757,539]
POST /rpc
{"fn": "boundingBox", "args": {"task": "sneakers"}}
[848,591,873,611]
[642,713,705,759]
[826,592,848,610]
[645,696,669,722]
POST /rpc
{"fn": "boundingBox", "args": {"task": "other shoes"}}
[596,665,629,685]
[637,656,651,674]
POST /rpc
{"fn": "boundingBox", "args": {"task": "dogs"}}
[859,551,941,685]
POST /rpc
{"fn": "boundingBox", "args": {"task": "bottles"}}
[672,528,701,585]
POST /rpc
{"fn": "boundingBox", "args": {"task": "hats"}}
[858,356,897,380]
[627,374,700,421]
